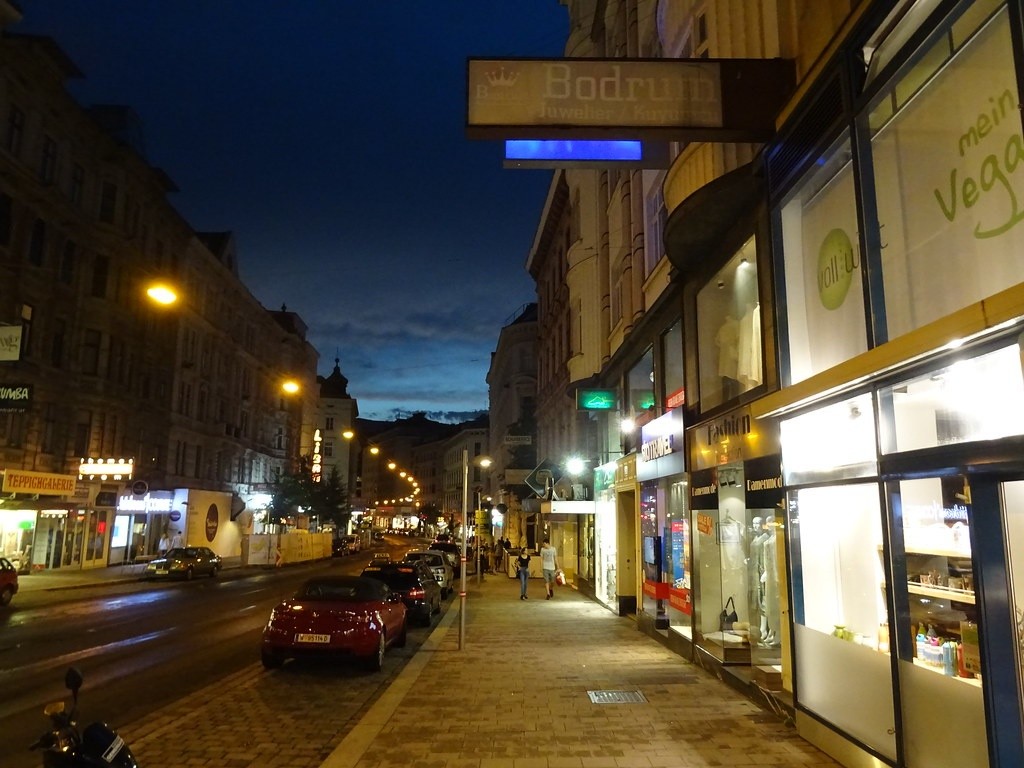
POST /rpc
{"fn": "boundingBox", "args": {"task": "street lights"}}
[476,490,492,585]
[458,450,493,651]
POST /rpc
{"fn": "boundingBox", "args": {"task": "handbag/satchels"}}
[555,569,566,586]
[719,597,738,630]
[514,554,522,567]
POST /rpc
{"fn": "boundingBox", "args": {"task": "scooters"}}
[28,665,140,768]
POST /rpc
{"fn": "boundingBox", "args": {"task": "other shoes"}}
[547,595,550,600]
[524,594,528,599]
[550,590,553,597]
[521,595,525,600]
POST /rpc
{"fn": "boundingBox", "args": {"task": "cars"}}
[402,549,457,600]
[0,556,19,607]
[427,540,460,579]
[144,547,223,581]
[260,574,410,670]
[384,526,452,543]
[359,551,443,629]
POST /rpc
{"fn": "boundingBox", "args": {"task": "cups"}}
[919,574,929,583]
[964,577,974,591]
[948,577,964,589]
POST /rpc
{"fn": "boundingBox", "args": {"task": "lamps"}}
[734,471,742,488]
[718,471,727,488]
[740,250,749,263]
[718,279,725,289]
[648,404,677,412]
[726,470,736,488]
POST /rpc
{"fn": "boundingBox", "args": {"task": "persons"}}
[472,536,511,577]
[159,533,169,555]
[171,531,184,548]
[540,538,560,600]
[516,547,531,600]
[444,531,456,545]
[751,517,781,645]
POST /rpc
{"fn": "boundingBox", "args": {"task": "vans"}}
[342,534,361,553]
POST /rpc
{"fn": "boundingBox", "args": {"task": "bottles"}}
[910,622,975,679]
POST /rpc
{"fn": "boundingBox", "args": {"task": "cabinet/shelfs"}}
[877,547,982,680]
